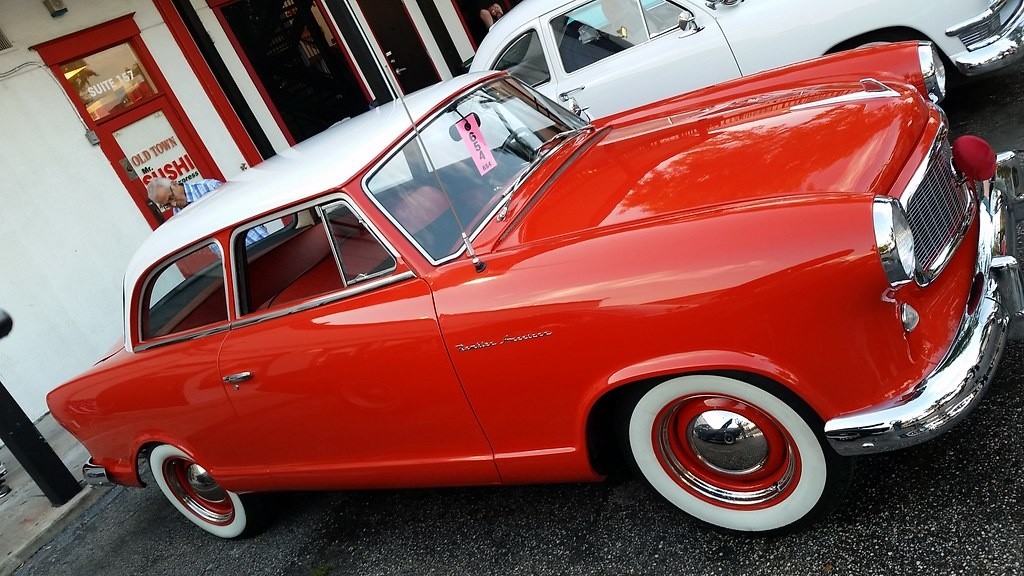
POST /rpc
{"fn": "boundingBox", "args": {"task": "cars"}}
[324,0,1023,208]
[45,40,1023,541]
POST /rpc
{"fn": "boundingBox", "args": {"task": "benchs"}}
[148,216,362,337]
[258,185,465,314]
[509,56,549,87]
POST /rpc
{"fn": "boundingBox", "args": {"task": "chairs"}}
[601,0,666,40]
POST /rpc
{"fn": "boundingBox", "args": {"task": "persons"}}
[147,176,270,260]
[480,2,504,28]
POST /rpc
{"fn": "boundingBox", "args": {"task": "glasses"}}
[164,185,175,210]
